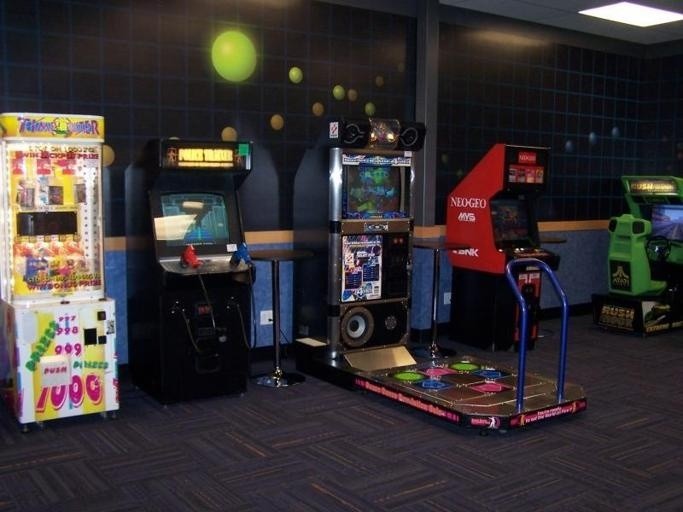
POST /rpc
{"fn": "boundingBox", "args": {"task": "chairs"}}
[606,213,668,296]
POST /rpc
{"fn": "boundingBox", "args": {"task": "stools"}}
[248,249,310,389]
[414,241,471,362]
[538,237,567,337]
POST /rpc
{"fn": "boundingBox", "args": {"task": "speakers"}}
[338,296,410,353]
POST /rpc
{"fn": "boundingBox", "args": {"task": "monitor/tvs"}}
[648,204,683,243]
[157,193,229,248]
[490,199,530,243]
[344,165,408,220]
